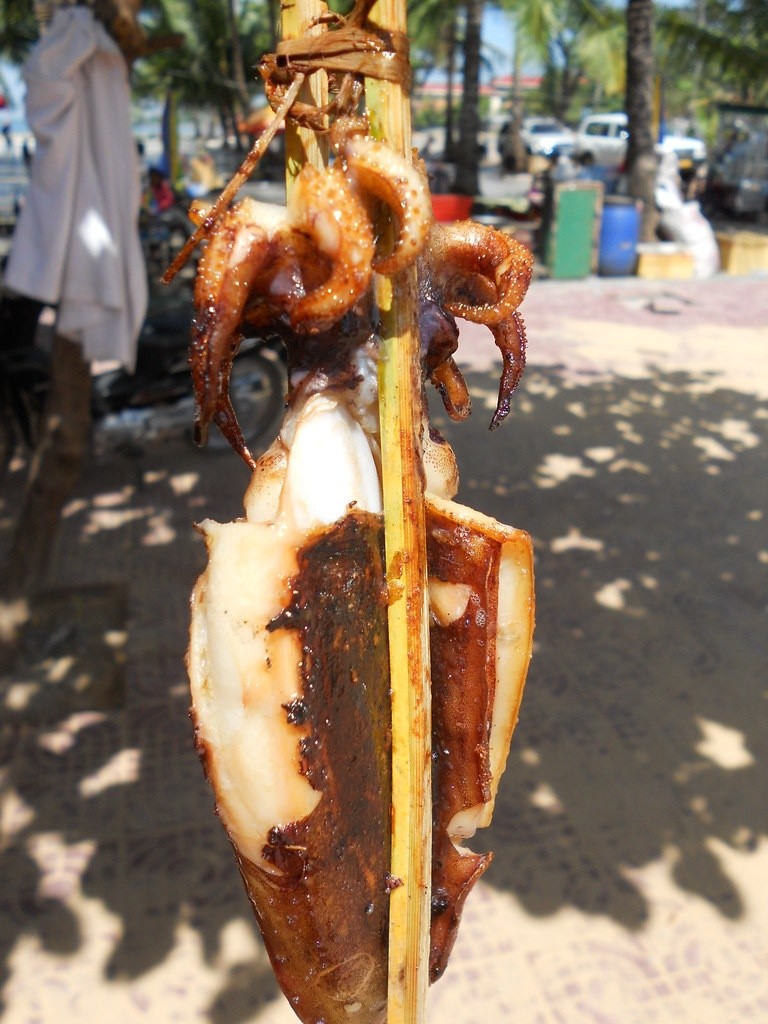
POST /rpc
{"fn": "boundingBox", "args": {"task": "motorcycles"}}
[0,189,311,466]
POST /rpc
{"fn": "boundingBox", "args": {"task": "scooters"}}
[420,133,486,169]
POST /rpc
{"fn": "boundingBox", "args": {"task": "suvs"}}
[572,114,706,182]
[496,118,584,168]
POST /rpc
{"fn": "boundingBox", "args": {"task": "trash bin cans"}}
[543,179,605,281]
